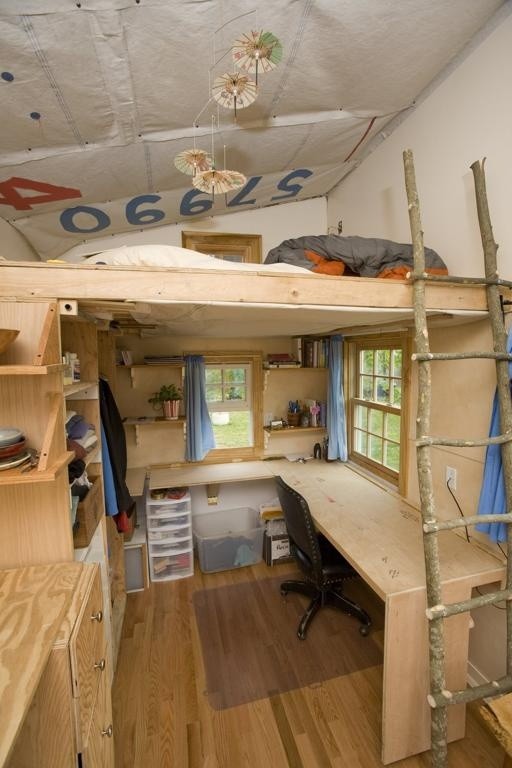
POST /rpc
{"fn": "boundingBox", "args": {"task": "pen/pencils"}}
[294,400,300,414]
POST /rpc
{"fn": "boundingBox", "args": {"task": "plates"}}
[0,427,32,472]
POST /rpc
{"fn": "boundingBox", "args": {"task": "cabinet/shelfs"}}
[116,362,187,449]
[145,487,195,583]
[261,367,328,450]
[0,561,114,768]
[56,310,127,684]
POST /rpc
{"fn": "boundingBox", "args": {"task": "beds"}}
[0,257,512,341]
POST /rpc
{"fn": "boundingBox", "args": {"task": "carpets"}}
[193,571,383,711]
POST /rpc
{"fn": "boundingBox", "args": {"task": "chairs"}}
[272,475,373,641]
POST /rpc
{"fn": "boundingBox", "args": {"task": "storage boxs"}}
[192,506,266,574]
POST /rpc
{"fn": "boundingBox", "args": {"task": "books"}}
[143,353,185,367]
[260,333,330,369]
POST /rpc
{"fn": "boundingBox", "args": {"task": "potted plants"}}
[148,383,184,421]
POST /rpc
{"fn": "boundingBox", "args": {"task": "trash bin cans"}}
[262,510,297,567]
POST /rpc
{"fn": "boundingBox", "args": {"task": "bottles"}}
[313,443,321,460]
[62,352,80,385]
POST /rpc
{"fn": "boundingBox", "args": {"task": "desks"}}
[149,455,510,765]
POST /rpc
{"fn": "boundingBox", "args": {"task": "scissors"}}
[289,401,297,413]
